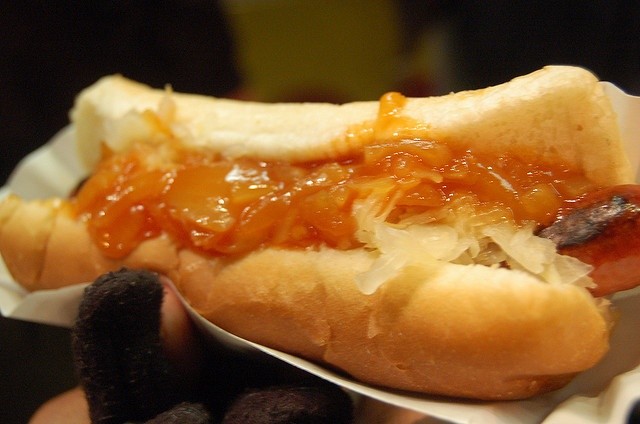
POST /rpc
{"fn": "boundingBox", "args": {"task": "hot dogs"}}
[1,68,639,400]
